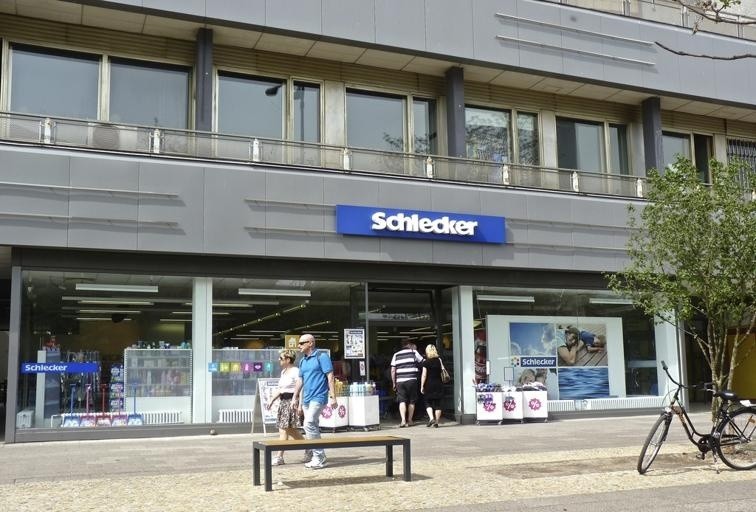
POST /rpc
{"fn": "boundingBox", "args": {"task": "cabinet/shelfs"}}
[34,349,330,427]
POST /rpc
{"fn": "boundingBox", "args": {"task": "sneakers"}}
[305,454,327,468]
[302,448,313,463]
[272,457,284,465]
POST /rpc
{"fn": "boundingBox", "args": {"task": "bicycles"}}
[636,359,755,477]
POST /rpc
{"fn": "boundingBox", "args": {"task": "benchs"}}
[253,435,411,492]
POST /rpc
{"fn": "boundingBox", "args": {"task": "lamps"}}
[589,298,633,305]
[62,283,310,322]
[476,294,535,302]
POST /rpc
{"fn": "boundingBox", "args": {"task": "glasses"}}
[280,356,287,360]
[299,341,314,345]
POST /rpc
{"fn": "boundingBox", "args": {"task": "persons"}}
[559,324,607,353]
[389,338,426,427]
[330,351,351,384]
[557,325,584,366]
[518,368,557,400]
[288,334,339,469]
[266,350,313,466]
[419,343,451,428]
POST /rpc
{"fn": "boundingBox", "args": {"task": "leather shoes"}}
[428,420,438,428]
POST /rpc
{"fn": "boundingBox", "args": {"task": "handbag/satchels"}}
[438,357,451,384]
